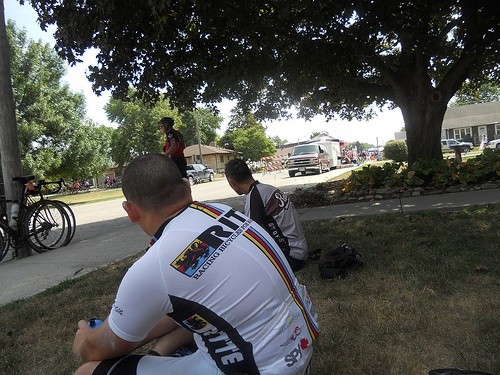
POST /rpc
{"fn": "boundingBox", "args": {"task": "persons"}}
[67,178,94,194]
[157,117,189,179]
[72,153,319,375]
[103,176,120,188]
[261,154,287,175]
[343,150,385,164]
[24,180,35,197]
[225,158,309,272]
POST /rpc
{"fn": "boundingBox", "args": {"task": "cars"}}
[186,163,216,185]
[441,139,474,153]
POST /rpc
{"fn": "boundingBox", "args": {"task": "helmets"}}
[157,117,174,125]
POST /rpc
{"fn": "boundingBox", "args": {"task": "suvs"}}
[488,138,500,150]
[287,144,331,177]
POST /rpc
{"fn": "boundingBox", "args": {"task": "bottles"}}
[11,202,19,227]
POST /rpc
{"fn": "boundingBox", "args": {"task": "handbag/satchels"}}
[318,245,364,278]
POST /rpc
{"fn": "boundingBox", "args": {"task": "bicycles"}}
[0,175,76,262]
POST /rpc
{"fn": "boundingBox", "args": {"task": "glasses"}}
[158,126,163,128]
